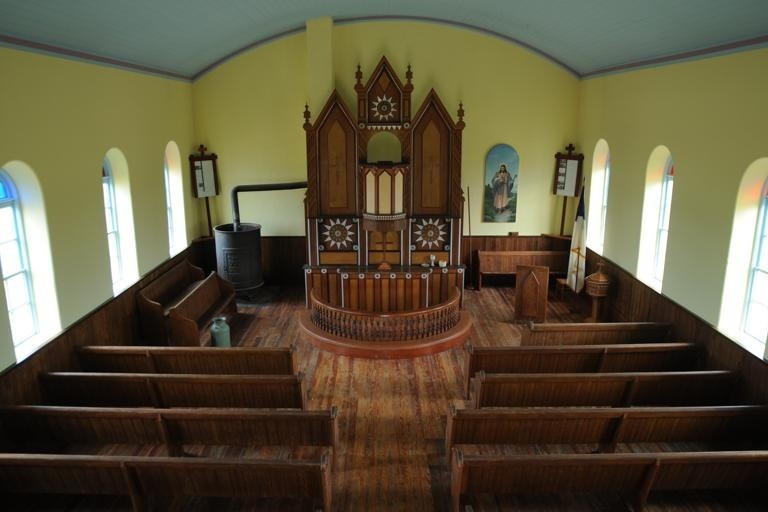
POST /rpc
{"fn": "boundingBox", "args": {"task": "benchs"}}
[475,249,568,294]
[137,257,205,335]
[166,269,240,346]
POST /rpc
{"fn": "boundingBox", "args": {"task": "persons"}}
[491,163,514,214]
